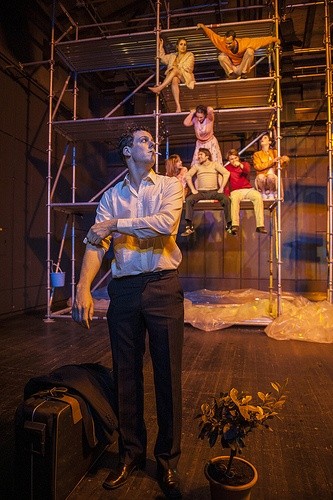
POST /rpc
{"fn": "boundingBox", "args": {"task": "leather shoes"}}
[157,459,177,488]
[103,459,145,489]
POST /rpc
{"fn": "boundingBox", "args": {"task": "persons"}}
[148,37,196,113]
[196,22,281,80]
[165,104,290,237]
[74,123,185,498]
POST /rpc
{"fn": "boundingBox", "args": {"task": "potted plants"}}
[192,378,289,500]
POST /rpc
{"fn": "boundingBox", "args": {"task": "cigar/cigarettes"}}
[151,150,162,156]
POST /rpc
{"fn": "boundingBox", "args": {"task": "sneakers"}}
[180,224,195,236]
[233,225,239,232]
[256,227,267,233]
[225,226,236,236]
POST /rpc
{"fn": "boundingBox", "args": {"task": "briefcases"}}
[14,363,117,500]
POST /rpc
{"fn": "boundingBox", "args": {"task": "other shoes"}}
[268,193,274,199]
[262,194,267,199]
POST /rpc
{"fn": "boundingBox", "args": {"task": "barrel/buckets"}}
[50,264,66,287]
